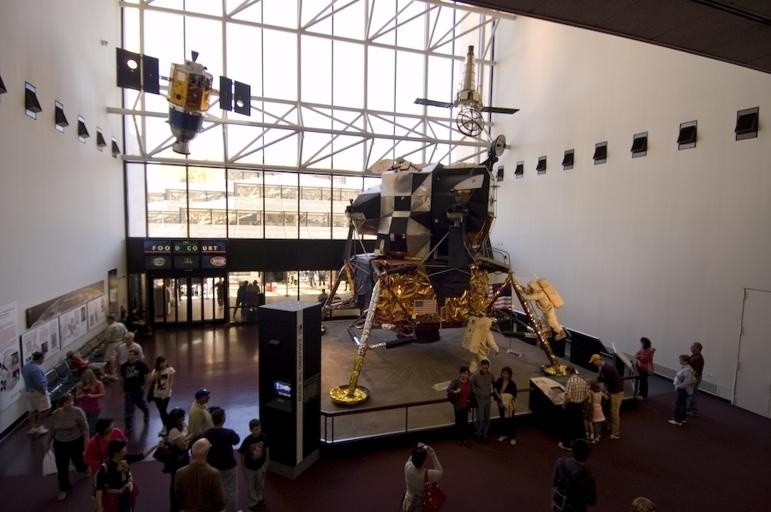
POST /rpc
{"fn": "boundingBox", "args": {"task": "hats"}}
[588,353,599,364]
[96,417,114,431]
[195,388,211,398]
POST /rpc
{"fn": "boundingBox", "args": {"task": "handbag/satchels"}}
[147,369,157,402]
[152,427,175,462]
[423,468,448,511]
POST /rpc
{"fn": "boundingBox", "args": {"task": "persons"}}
[667,355,696,425]
[634,336,656,400]
[173,437,229,512]
[471,359,496,442]
[551,439,597,511]
[116,333,145,377]
[446,366,474,447]
[161,408,191,503]
[213,277,225,307]
[82,416,129,474]
[688,342,705,419]
[120,350,152,434]
[43,392,91,500]
[188,388,214,436]
[559,364,588,453]
[125,442,165,464]
[148,355,176,435]
[204,408,242,512]
[587,376,610,441]
[100,313,129,381]
[234,280,261,318]
[239,418,270,507]
[93,439,138,512]
[76,369,105,427]
[589,354,624,440]
[630,497,656,512]
[493,367,519,446]
[320,289,328,302]
[22,352,53,436]
[400,444,445,512]
[64,350,119,383]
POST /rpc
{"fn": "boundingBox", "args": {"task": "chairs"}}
[54,360,81,391]
[42,369,69,402]
[77,329,110,362]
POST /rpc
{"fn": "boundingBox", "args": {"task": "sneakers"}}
[56,491,67,502]
[28,425,36,435]
[669,418,681,426]
[635,394,647,400]
[596,436,600,442]
[608,433,621,440]
[82,468,90,480]
[158,424,168,436]
[587,437,595,442]
[34,425,49,434]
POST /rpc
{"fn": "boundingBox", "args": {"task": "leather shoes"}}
[464,439,471,448]
[142,409,150,423]
[497,435,508,444]
[511,439,517,447]
[558,441,570,450]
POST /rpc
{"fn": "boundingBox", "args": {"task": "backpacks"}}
[162,423,189,474]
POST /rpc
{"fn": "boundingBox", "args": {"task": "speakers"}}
[257,301,322,467]
